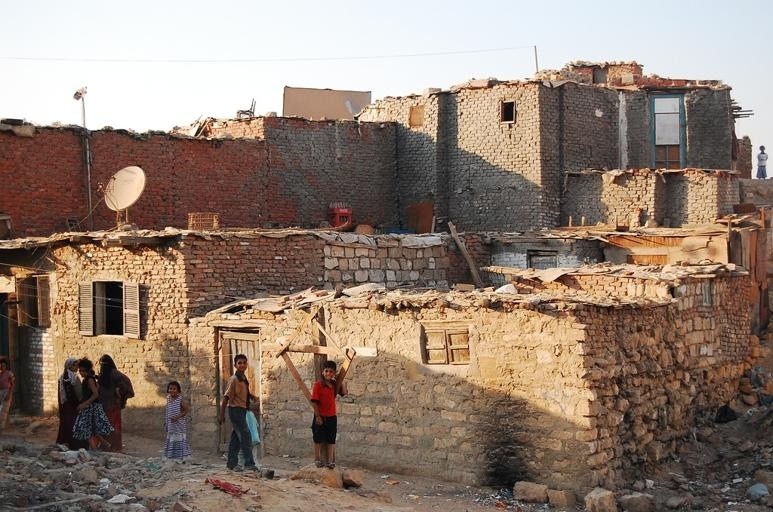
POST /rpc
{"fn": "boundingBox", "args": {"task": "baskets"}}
[188,212,220,231]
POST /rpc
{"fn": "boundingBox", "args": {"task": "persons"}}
[71,359,114,451]
[756,146,768,181]
[310,360,348,469]
[162,379,191,459]
[94,354,135,451]
[54,357,81,451]
[217,353,260,471]
[0,358,14,429]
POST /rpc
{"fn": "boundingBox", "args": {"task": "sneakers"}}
[97,440,112,451]
[227,464,258,472]
[315,459,336,469]
